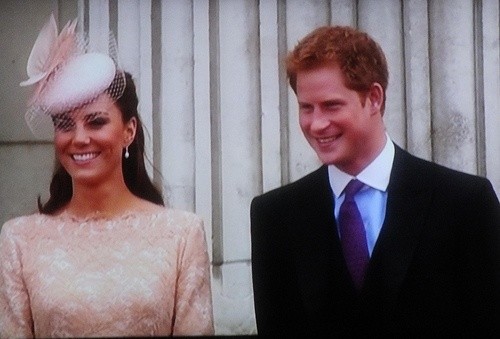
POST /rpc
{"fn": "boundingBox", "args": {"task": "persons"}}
[250,22,500,338]
[0,60,218,338]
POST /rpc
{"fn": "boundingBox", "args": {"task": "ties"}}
[337,178,372,296]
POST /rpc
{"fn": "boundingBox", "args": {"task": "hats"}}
[18,12,127,141]
